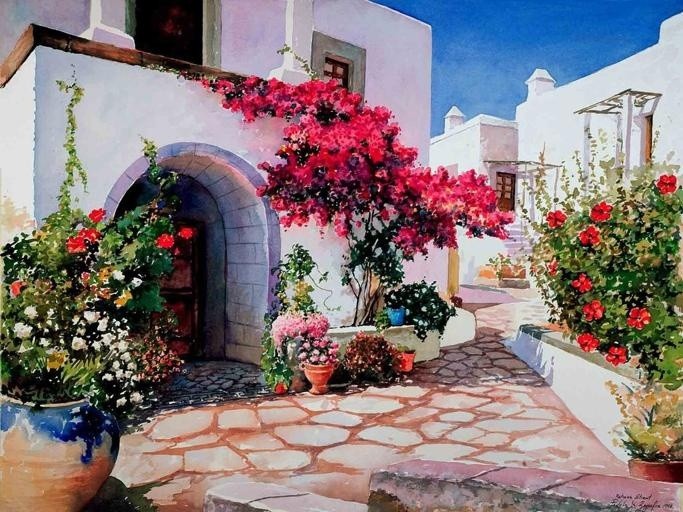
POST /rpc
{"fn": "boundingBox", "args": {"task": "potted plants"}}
[385,294,405,326]
[266,354,291,396]
[396,344,414,373]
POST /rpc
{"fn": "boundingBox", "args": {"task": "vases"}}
[626,455,682,482]
[286,361,308,394]
[0,392,120,511]
[300,362,339,395]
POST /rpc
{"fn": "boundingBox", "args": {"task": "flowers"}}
[606,380,681,457]
[271,314,327,368]
[296,339,341,365]
[0,197,192,412]
